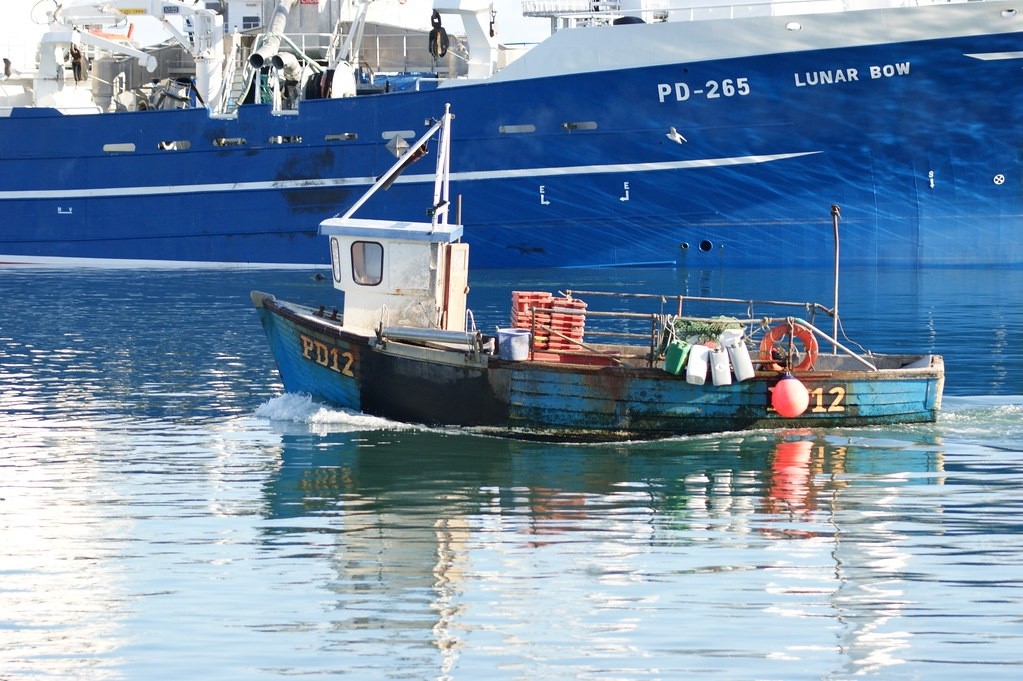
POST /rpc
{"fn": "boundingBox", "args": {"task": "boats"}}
[1,0,1023,270]
[249,96,949,445]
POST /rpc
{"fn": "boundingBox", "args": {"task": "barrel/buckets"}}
[663,341,755,386]
[497,328,531,361]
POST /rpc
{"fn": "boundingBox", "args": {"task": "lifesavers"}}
[759,322,819,371]
